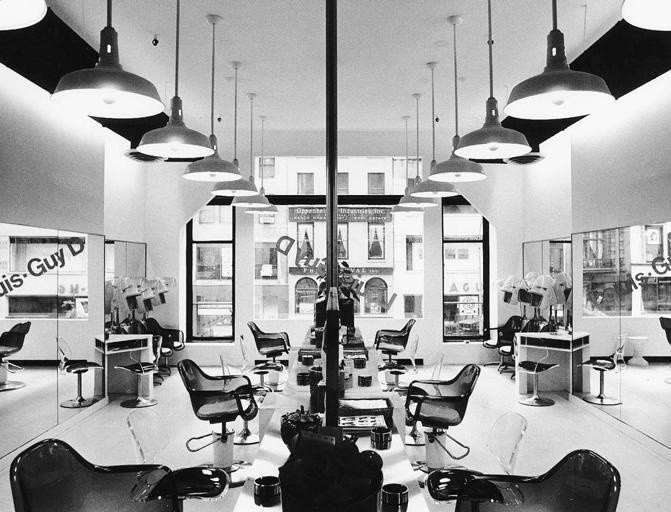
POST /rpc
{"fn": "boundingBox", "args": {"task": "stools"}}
[628,336,649,367]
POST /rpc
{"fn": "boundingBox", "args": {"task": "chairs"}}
[9,438,183,512]
[56,337,104,409]
[0,320,31,391]
[113,316,185,408]
[177,320,291,452]
[659,317,671,344]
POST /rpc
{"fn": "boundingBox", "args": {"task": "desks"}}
[231,324,429,512]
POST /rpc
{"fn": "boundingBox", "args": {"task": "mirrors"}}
[571,227,621,421]
[620,221,671,449]
[57,230,106,426]
[105,239,147,328]
[520,237,572,331]
[0,222,58,460]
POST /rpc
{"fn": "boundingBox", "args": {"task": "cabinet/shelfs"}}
[93,337,104,395]
